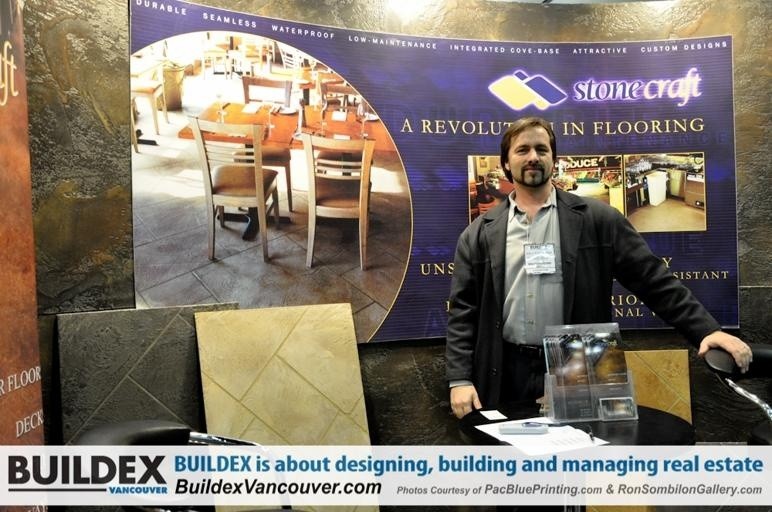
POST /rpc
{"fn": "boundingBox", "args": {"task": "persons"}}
[445,116,753,419]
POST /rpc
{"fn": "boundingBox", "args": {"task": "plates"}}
[365,113,379,121]
[278,108,296,114]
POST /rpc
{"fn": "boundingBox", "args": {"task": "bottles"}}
[643,173,647,183]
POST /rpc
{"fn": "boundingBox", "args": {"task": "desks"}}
[175,99,403,240]
[457,399,695,512]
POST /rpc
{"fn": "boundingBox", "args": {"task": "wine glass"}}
[357,103,368,135]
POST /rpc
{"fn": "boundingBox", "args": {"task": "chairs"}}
[130,32,375,272]
